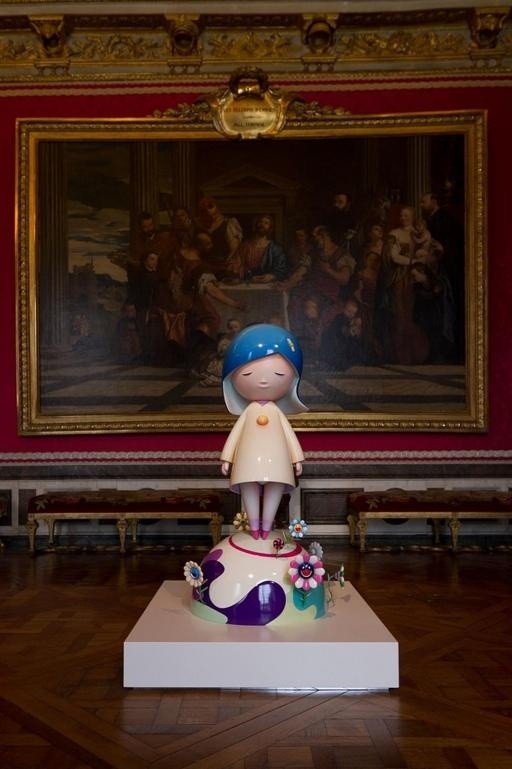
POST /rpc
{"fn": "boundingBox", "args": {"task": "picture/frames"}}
[208,280,290,338]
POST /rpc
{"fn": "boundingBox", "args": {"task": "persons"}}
[219,322,306,541]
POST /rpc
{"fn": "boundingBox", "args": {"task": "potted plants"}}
[15,105,493,435]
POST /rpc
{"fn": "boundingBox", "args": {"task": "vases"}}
[183,511,345,613]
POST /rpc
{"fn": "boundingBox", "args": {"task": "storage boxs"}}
[345,487,512,553]
[24,489,224,556]
